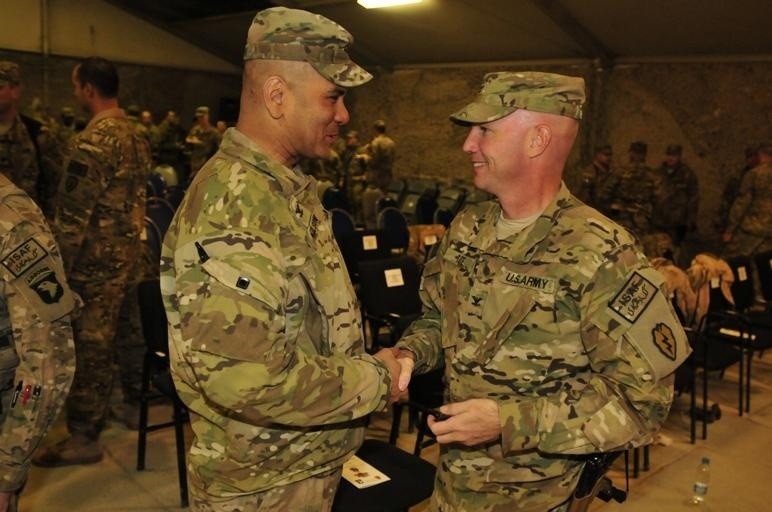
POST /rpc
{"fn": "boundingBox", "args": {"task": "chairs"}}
[137,175,770,512]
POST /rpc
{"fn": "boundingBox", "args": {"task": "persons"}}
[1,58,229,511]
[714,145,759,253]
[594,141,671,233]
[573,142,620,219]
[157,7,411,512]
[655,142,701,240]
[719,140,772,260]
[298,119,395,197]
[391,70,695,512]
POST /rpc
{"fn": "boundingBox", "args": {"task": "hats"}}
[374,120,385,128]
[196,106,208,116]
[346,130,359,138]
[243,7,373,88]
[62,108,75,118]
[746,141,772,157]
[596,143,681,155]
[449,71,586,127]
[0,61,20,85]
[128,105,140,116]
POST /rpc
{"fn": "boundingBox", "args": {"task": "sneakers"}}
[107,402,142,429]
[31,435,103,466]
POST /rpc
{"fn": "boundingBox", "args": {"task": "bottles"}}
[688,457,710,506]
